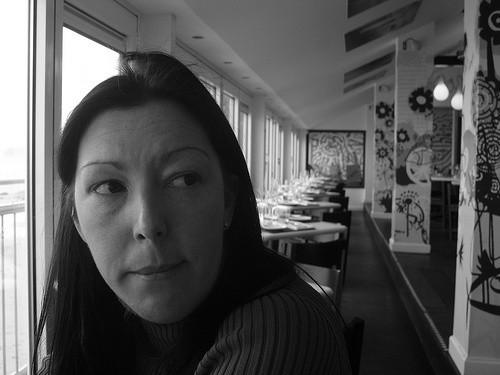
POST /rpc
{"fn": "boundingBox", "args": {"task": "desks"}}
[252,169,460,312]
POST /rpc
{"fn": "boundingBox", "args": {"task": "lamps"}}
[433,75,449,102]
[450,84,463,110]
[403,38,415,52]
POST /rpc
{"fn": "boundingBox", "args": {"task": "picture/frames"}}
[305,130,366,189]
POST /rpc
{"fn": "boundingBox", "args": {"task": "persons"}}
[29,49,355,374]
